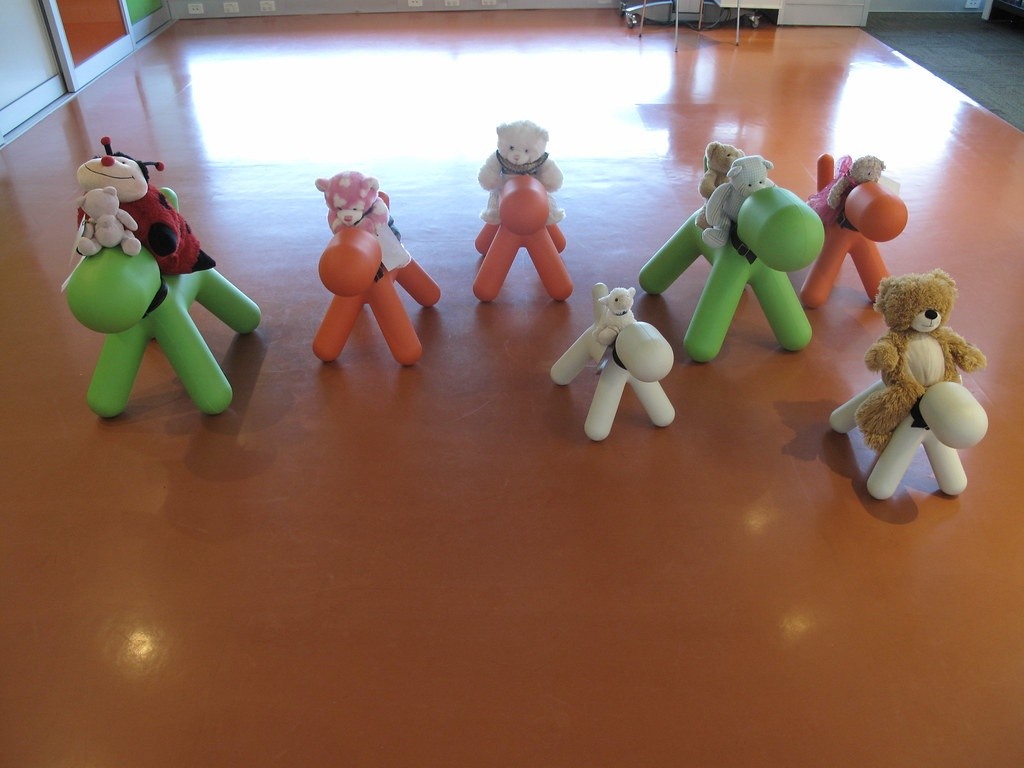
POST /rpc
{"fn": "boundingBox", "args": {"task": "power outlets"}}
[965,0,981,8]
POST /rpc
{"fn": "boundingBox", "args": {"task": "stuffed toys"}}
[314,171,389,233]
[477,119,564,226]
[694,141,748,230]
[700,155,778,249]
[591,288,635,346]
[826,155,885,209]
[854,269,986,453]
[75,136,216,277]
[74,186,140,256]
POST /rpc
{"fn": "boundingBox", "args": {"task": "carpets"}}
[861,0,1024,131]
